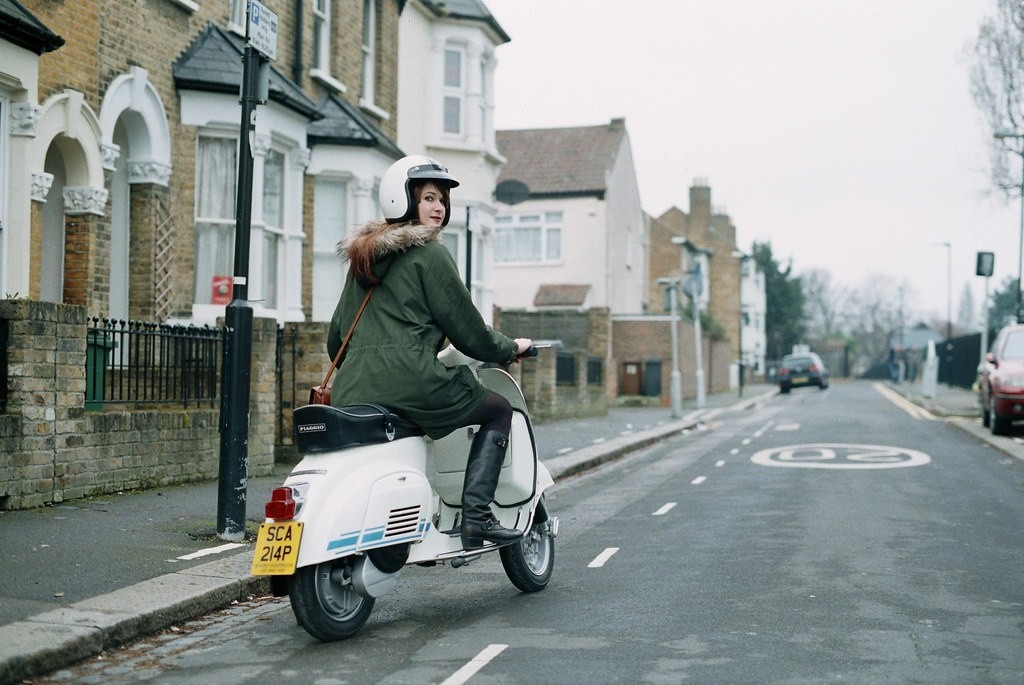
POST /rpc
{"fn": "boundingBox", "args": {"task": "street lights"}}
[671,233,717,413]
[974,249,996,380]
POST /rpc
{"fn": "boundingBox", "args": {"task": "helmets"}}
[378,154,460,228]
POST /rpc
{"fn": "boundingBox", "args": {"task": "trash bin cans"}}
[643,357,662,396]
[621,361,642,393]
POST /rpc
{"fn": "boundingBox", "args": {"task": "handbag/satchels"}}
[309,385,331,407]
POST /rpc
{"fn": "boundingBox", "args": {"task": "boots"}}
[461,429,523,552]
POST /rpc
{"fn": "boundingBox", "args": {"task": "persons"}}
[327,155,534,551]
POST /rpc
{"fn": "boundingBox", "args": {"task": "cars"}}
[777,338,828,393]
[978,323,1024,436]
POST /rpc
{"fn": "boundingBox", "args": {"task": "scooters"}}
[251,338,565,642]
